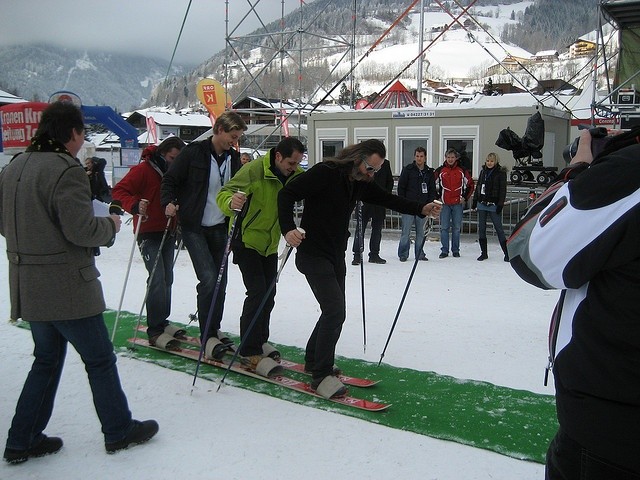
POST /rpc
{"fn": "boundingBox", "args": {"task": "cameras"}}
[562,126,609,165]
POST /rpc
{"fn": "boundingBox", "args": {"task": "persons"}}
[113,137,186,351]
[217,139,305,377]
[398,147,434,261]
[1,100,159,462]
[472,153,510,262]
[240,152,251,165]
[159,113,246,359]
[278,140,442,398]
[433,149,474,259]
[82,158,102,200]
[351,158,394,265]
[506,127,638,475]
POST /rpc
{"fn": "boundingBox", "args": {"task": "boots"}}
[477,238,488,261]
[500,240,509,261]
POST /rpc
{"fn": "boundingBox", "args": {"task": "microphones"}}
[109,199,125,215]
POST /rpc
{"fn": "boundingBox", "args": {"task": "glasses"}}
[363,160,381,172]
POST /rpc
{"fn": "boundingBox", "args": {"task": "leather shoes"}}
[3,437,63,464]
[104,419,159,455]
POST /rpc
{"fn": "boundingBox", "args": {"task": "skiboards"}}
[126,324,392,412]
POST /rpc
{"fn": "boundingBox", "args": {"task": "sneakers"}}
[453,253,460,257]
[149,333,174,348]
[311,377,346,396]
[305,363,339,375]
[368,252,386,264]
[199,332,234,358]
[236,343,280,370]
[439,252,447,258]
[352,252,361,265]
[163,324,184,337]
